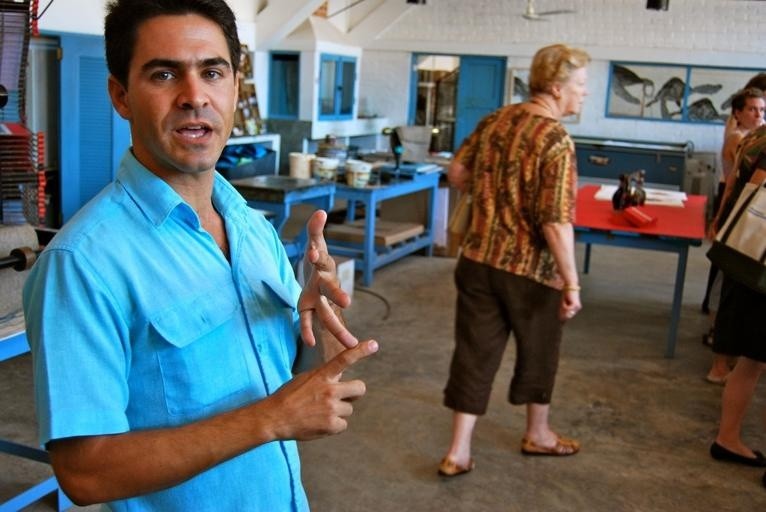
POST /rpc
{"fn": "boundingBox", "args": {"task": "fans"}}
[475,0,578,21]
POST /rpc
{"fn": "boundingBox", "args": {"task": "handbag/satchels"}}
[705,182,765,295]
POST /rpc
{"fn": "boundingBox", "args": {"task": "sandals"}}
[438,456,474,478]
[522,436,581,456]
[710,442,766,467]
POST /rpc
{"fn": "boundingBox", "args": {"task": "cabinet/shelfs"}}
[262,39,363,122]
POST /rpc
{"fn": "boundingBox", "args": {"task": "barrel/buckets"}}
[289,152,315,179]
[312,158,339,182]
[345,159,374,190]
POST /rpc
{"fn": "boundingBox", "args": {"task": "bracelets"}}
[561,284,581,293]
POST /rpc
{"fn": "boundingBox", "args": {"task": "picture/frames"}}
[507,67,582,124]
[682,65,766,125]
[605,61,690,123]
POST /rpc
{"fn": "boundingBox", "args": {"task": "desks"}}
[574,184,708,358]
[0,311,75,512]
[573,137,688,189]
[226,134,458,286]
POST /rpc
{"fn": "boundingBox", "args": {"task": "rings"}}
[565,311,575,319]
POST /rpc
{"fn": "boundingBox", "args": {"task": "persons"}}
[695,70,766,492]
[17,1,379,511]
[433,41,594,478]
